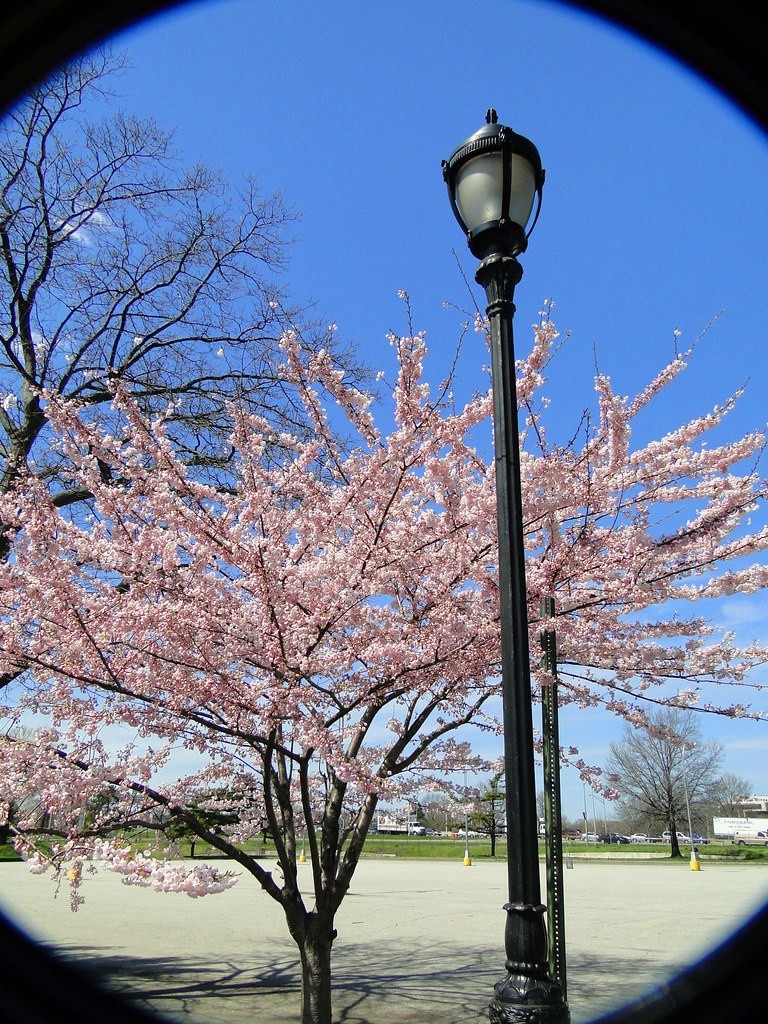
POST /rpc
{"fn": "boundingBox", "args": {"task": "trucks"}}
[377,815,426,836]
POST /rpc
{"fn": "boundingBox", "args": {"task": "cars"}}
[582,832,602,841]
[597,832,630,845]
[692,833,710,844]
[662,831,691,844]
[631,833,649,842]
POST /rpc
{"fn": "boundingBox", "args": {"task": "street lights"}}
[443,107,572,1024]
[588,794,607,834]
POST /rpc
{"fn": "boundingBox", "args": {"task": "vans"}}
[734,830,768,846]
